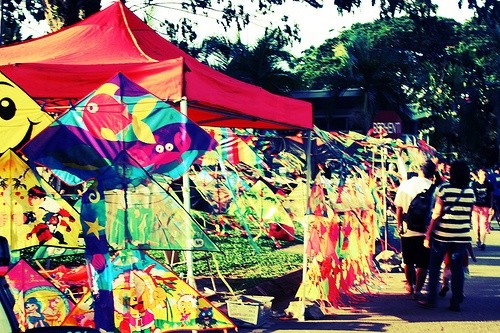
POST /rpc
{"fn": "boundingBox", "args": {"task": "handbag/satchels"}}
[426,214,441,237]
[395,183,437,234]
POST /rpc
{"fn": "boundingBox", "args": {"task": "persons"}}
[486,167,500,233]
[420,161,478,311]
[440,162,453,181]
[393,157,438,302]
[468,169,496,250]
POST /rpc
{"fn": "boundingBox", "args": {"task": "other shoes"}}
[418,296,438,307]
[450,298,463,312]
[479,243,487,250]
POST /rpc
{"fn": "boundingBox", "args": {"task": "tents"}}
[0,0,313,333]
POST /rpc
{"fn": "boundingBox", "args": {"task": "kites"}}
[0,66,453,333]
